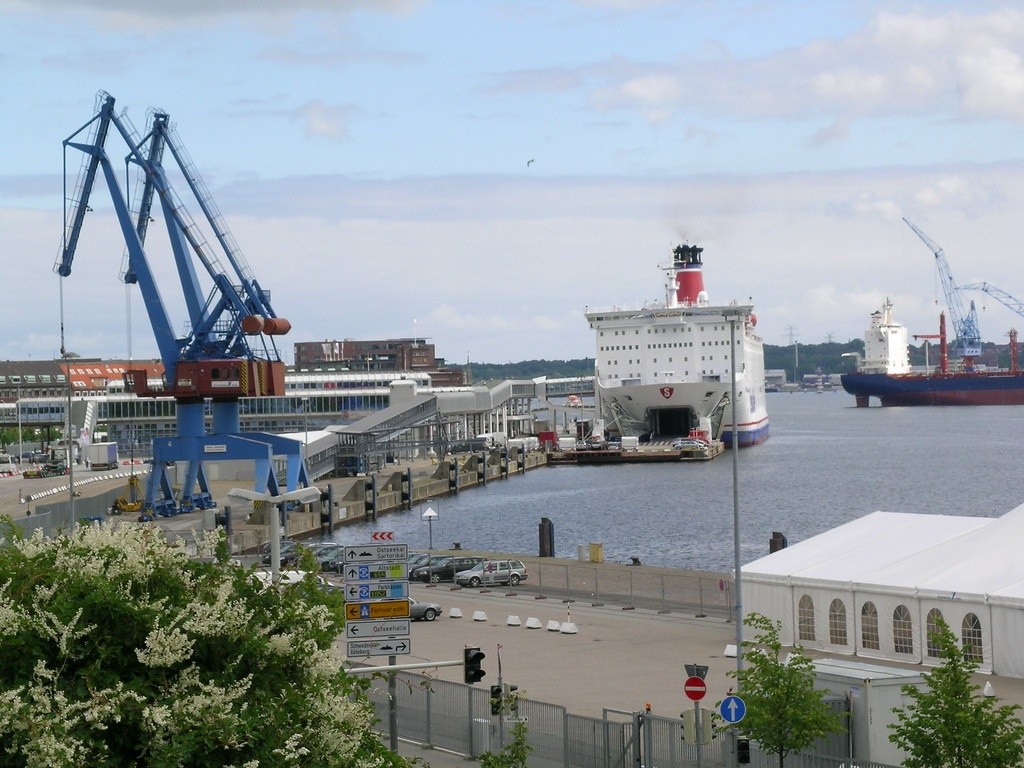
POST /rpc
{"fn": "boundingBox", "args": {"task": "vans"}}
[413,556,487,583]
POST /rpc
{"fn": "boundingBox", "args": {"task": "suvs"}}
[452,559,529,588]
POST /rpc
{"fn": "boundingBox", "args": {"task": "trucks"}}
[89,442,119,471]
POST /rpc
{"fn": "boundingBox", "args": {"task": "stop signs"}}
[684,675,707,701]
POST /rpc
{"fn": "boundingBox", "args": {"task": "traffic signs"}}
[343,545,411,658]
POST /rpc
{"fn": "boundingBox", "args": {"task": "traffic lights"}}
[738,739,750,764]
[508,685,519,712]
[464,647,486,683]
[491,686,502,715]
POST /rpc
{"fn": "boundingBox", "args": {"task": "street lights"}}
[723,311,748,694]
[301,397,310,466]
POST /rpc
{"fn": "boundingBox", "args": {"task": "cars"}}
[408,596,443,622]
[406,550,445,580]
[246,572,343,601]
[305,543,343,572]
[18,451,34,459]
[262,541,309,567]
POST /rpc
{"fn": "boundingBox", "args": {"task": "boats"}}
[841,296,1024,408]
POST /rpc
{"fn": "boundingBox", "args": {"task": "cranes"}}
[53,88,315,521]
[903,217,983,356]
